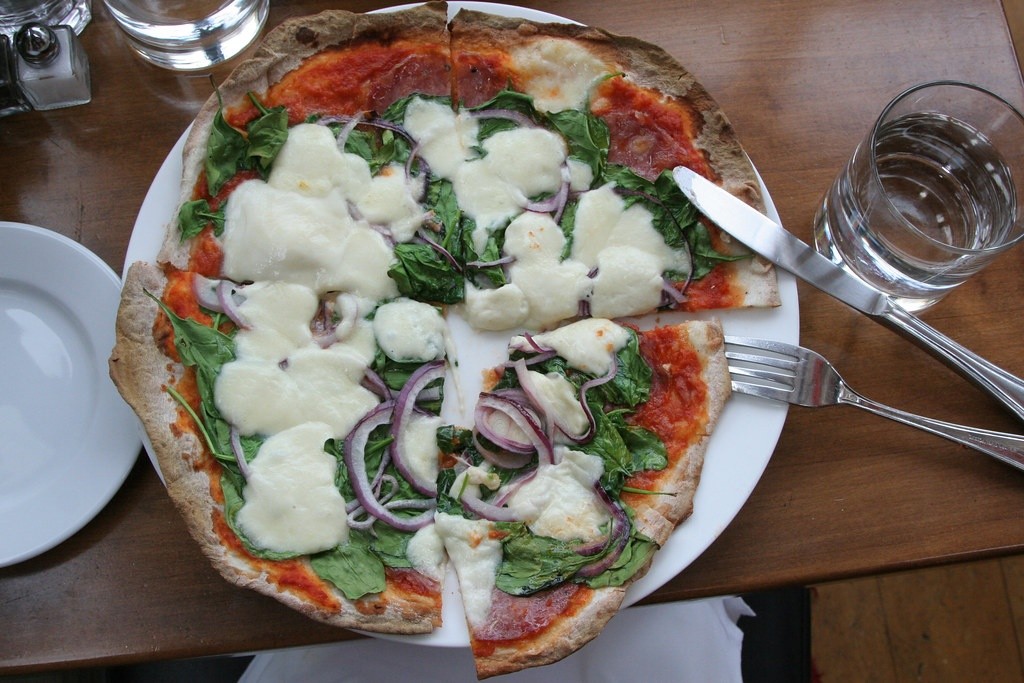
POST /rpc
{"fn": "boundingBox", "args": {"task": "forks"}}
[723,334,1024,472]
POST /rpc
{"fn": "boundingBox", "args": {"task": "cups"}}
[815,81,1022,317]
[103,0,270,70]
[0,0,93,41]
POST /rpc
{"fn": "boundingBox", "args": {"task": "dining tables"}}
[0,0,1024,676]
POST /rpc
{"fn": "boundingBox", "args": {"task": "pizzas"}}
[108,0,781,682]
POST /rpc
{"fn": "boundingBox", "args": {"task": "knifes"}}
[674,164,1024,431]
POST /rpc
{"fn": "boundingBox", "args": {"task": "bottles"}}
[13,17,92,109]
[0,32,26,115]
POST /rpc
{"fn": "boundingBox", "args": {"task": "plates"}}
[120,5,803,650]
[1,220,148,570]
